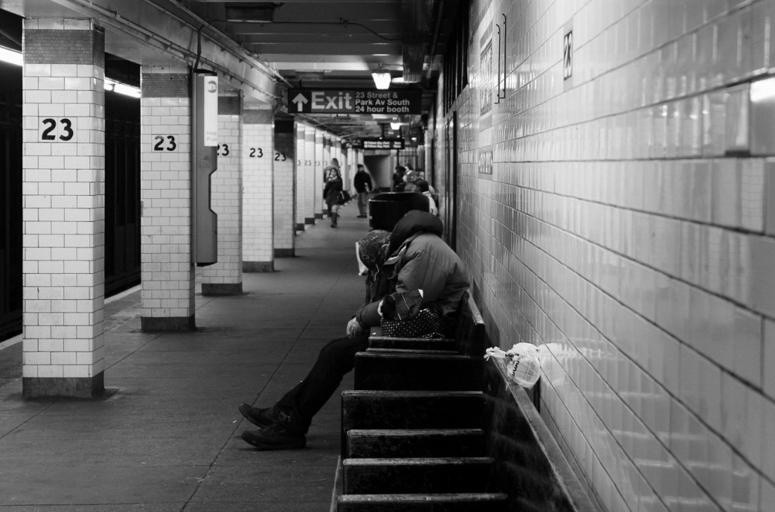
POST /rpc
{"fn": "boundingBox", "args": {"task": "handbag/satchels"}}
[335,190,352,206]
[378,294,444,340]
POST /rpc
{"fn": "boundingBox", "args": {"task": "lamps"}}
[370,63,391,89]
[222,1,285,24]
[390,116,401,131]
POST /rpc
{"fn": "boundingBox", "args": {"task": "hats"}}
[357,228,392,271]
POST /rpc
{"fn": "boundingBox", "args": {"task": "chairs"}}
[326,191,512,512]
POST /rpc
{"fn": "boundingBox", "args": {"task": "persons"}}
[322,168,343,228]
[352,162,374,220]
[235,208,472,453]
[390,162,439,217]
[324,157,342,218]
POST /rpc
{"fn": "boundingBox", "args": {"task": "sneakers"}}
[330,222,338,228]
[236,402,278,428]
[356,213,368,218]
[240,426,307,452]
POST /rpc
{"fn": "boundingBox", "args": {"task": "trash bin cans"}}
[368,192,430,232]
